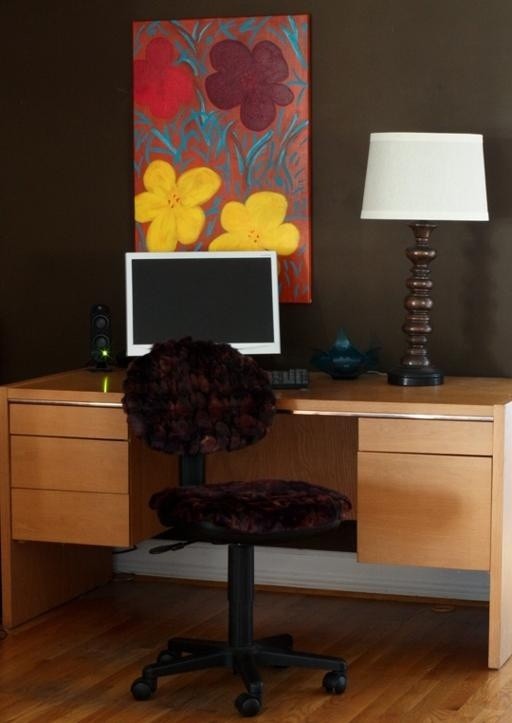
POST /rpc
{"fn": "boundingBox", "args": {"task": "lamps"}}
[360,132,492,387]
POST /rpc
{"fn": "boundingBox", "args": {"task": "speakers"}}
[88,304,113,373]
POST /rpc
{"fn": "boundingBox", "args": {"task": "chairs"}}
[120,336,353,718]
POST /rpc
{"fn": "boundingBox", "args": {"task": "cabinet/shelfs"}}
[357,415,495,570]
[2,402,178,548]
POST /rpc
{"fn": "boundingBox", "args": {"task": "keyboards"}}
[264,368,310,390]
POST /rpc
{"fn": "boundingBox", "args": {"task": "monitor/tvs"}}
[124,250,283,358]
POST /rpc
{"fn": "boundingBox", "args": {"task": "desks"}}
[1,367,511,669]
